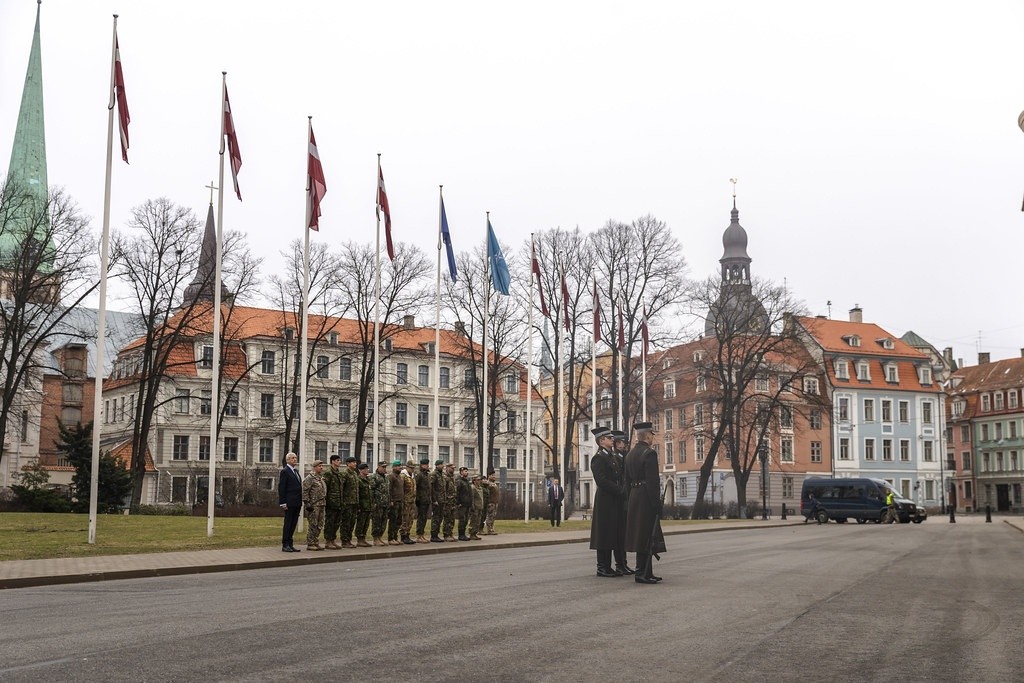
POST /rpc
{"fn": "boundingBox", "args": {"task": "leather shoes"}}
[282,546,293,552]
[626,566,636,573]
[290,545,301,551]
[616,567,632,575]
[606,568,623,576]
[597,570,616,577]
[636,578,657,584]
[651,576,662,581]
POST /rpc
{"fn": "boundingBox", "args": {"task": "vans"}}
[800,477,917,523]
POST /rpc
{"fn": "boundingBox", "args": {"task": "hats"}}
[590,426,614,438]
[312,460,323,467]
[611,430,627,440]
[330,455,495,480]
[632,422,656,435]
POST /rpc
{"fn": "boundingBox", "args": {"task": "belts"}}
[630,480,647,487]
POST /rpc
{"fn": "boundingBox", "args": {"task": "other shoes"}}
[307,545,318,551]
[316,544,325,550]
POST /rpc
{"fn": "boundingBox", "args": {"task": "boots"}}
[331,539,342,549]
[357,529,498,546]
[342,541,357,548]
[325,539,336,549]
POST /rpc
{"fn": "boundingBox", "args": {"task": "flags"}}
[115,34,131,165]
[593,280,601,342]
[441,198,458,282]
[561,264,571,331]
[488,221,512,295]
[224,86,243,201]
[376,167,394,261]
[618,300,625,351]
[308,127,327,232]
[642,307,650,354]
[533,244,550,316]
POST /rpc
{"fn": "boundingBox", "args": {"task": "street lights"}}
[759,448,768,520]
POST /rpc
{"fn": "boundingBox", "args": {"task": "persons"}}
[302,455,500,551]
[623,421,667,584]
[591,427,635,577]
[278,452,302,553]
[548,479,564,527]
[804,494,821,525]
[881,489,900,524]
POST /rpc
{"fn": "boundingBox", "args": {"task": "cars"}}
[913,506,927,523]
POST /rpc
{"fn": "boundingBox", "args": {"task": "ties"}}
[293,469,298,479]
[554,486,557,497]
